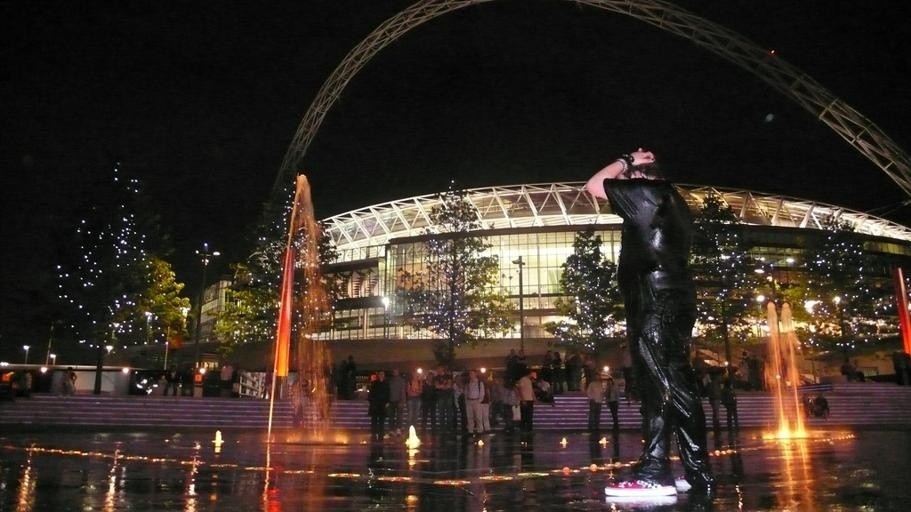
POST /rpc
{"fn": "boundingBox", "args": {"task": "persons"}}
[162,364,179,396]
[803,392,814,419]
[219,359,235,398]
[583,138,716,497]
[744,353,764,391]
[813,392,832,423]
[703,372,724,426]
[722,376,741,432]
[59,367,78,396]
[841,357,867,383]
[261,367,273,400]
[332,348,633,437]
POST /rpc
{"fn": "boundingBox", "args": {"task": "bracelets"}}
[616,153,635,174]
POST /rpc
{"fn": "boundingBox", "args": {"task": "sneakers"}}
[605,480,678,497]
[674,476,691,492]
[606,497,677,511]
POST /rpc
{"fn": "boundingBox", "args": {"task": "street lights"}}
[381,295,390,338]
[48,353,56,365]
[20,345,30,363]
[510,255,527,358]
[750,255,802,386]
[190,240,222,368]
